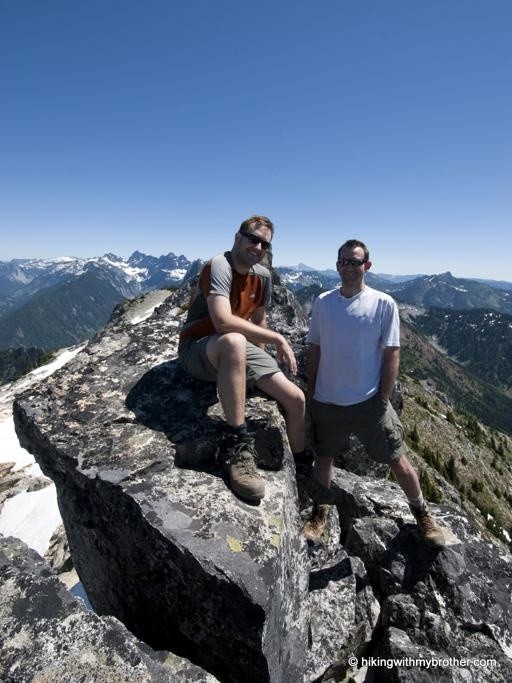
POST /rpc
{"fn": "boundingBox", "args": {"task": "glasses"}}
[338,258,366,267]
[238,230,270,249]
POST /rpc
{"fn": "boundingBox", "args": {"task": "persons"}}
[174,212,309,505]
[302,238,446,544]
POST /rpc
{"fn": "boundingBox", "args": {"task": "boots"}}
[295,453,315,508]
[218,427,265,500]
[302,491,330,545]
[408,501,445,548]
